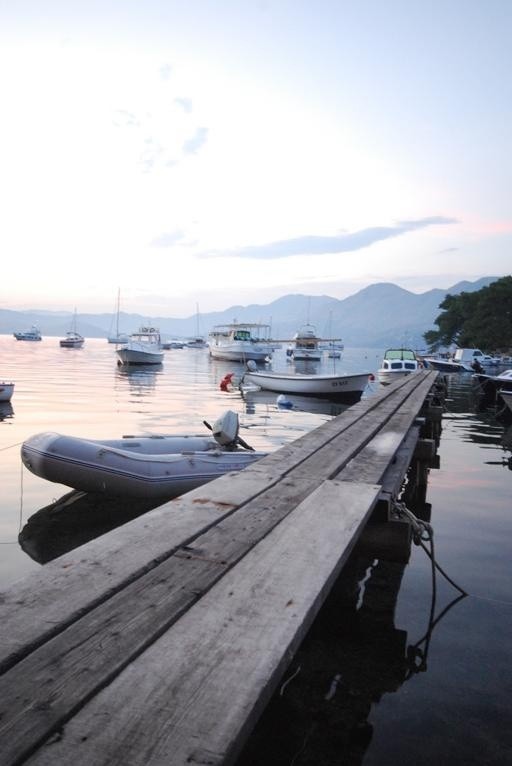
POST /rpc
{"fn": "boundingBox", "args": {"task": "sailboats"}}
[61,309,85,347]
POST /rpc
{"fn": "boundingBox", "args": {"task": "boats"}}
[239,387,353,419]
[19,488,170,566]
[15,319,43,342]
[0,383,14,402]
[0,400,16,422]
[22,411,275,502]
